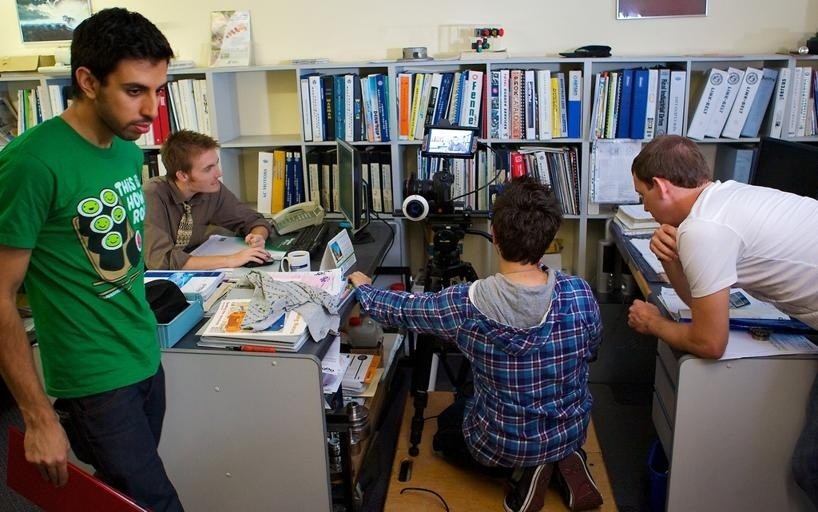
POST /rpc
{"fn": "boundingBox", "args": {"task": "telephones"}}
[271,201,325,235]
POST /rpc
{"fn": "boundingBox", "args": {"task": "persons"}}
[0,7,184,512]
[630,140,818,512]
[143,130,273,270]
[346,173,603,512]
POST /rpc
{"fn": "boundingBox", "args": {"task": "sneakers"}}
[502,461,543,512]
[556,447,605,511]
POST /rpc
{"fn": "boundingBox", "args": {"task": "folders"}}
[721,67,764,140]
[687,68,728,141]
[741,67,779,138]
[705,67,745,139]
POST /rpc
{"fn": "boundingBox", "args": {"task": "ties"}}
[172,201,195,253]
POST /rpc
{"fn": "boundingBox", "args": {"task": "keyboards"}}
[284,221,329,262]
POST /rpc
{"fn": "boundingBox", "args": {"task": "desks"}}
[142,219,400,512]
[610,219,817,511]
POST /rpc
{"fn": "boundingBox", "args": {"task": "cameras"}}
[402,123,478,265]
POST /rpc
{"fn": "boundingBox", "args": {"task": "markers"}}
[225,344,274,353]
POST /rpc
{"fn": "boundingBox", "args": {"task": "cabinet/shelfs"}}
[0,53,817,386]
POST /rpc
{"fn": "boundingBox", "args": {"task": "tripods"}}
[409,261,486,456]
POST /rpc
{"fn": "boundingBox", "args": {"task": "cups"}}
[281,251,310,273]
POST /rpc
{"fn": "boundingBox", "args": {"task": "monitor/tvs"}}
[337,137,375,244]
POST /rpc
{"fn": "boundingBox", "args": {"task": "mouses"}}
[243,257,274,268]
[398,460,411,482]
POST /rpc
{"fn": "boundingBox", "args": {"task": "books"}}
[397,68,486,139]
[611,203,662,235]
[405,145,582,217]
[299,66,389,142]
[9,79,216,150]
[143,261,344,353]
[320,347,386,403]
[590,68,688,139]
[488,67,584,139]
[254,150,394,214]
[591,142,642,204]
[688,65,818,138]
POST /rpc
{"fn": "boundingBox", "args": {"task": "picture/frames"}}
[17,0,95,42]
[615,0,709,21]
